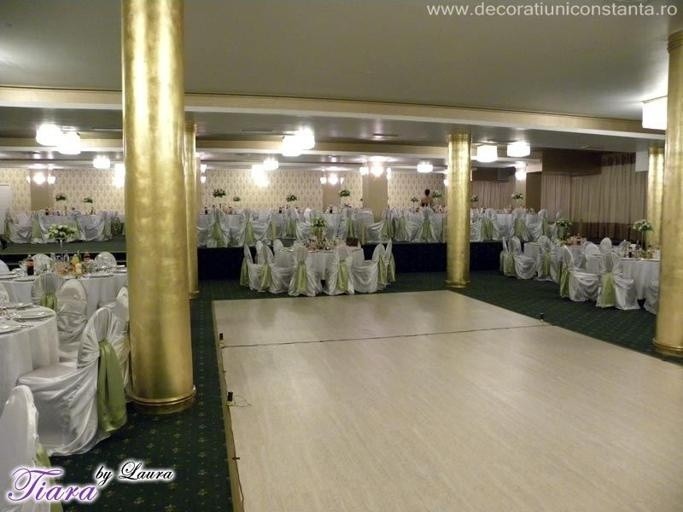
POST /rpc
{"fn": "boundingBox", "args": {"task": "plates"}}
[0,302,53,334]
[0,271,35,281]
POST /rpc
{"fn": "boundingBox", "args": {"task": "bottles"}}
[26,255,34,275]
[64,250,90,273]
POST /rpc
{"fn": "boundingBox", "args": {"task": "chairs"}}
[194,204,446,297]
[0,206,132,511]
[468,205,663,315]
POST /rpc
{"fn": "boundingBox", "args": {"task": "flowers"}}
[55,193,66,201]
[212,188,226,197]
[285,193,297,201]
[338,188,350,196]
[472,192,479,202]
[431,190,441,197]
[511,191,523,199]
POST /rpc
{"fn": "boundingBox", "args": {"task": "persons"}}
[420,189,433,207]
